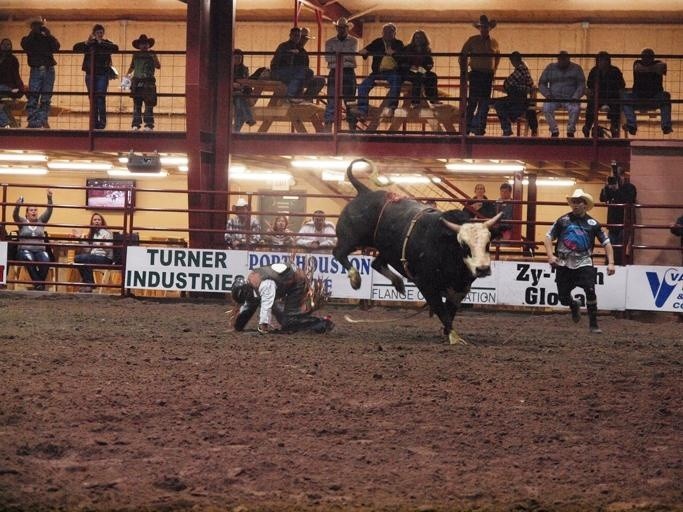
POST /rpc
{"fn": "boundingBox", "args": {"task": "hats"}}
[24,15,48,27]
[472,15,497,30]
[229,275,249,306]
[131,34,155,49]
[331,17,354,32]
[297,26,317,42]
[564,188,594,213]
[229,198,249,214]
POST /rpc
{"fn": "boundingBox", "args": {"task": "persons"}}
[670,218,683,236]
[544,188,616,333]
[256,215,295,253]
[73,213,114,293]
[492,183,513,247]
[223,262,336,334]
[224,198,262,250]
[600,167,637,265]
[294,210,338,254]
[12,188,53,290]
[463,184,495,218]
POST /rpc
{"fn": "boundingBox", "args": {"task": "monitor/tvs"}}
[86,178,135,211]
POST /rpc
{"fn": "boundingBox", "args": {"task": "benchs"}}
[0,96,73,128]
[228,73,670,139]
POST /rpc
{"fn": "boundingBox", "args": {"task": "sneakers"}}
[611,131,619,139]
[231,94,444,132]
[566,133,574,137]
[499,130,514,137]
[663,127,673,135]
[1,122,154,132]
[465,127,486,137]
[581,125,589,137]
[550,133,560,138]
[621,124,637,137]
[29,281,96,294]
[530,128,538,138]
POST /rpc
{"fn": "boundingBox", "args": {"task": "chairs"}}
[7,230,186,297]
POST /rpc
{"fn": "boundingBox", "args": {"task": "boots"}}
[585,300,603,335]
[560,296,582,324]
[285,316,336,334]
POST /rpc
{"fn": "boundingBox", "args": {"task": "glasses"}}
[570,198,585,205]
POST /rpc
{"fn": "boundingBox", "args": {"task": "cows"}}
[329,157,506,347]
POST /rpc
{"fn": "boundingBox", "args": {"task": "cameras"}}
[608,159,619,185]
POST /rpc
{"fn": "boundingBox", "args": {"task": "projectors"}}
[127,156,162,174]
